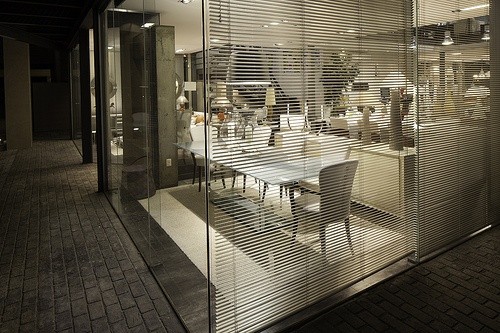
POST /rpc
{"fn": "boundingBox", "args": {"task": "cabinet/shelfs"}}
[334,136,415,219]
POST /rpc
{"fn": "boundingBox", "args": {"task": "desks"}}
[173,137,333,235]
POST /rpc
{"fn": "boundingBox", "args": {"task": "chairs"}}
[288,143,351,213]
[188,105,391,202]
[292,159,360,258]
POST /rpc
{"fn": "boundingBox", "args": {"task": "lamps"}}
[442,31,454,45]
[409,36,416,48]
[482,33,490,40]
[225,51,273,84]
[177,96,188,105]
[265,84,276,105]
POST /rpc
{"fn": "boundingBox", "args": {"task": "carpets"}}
[138,172,405,309]
